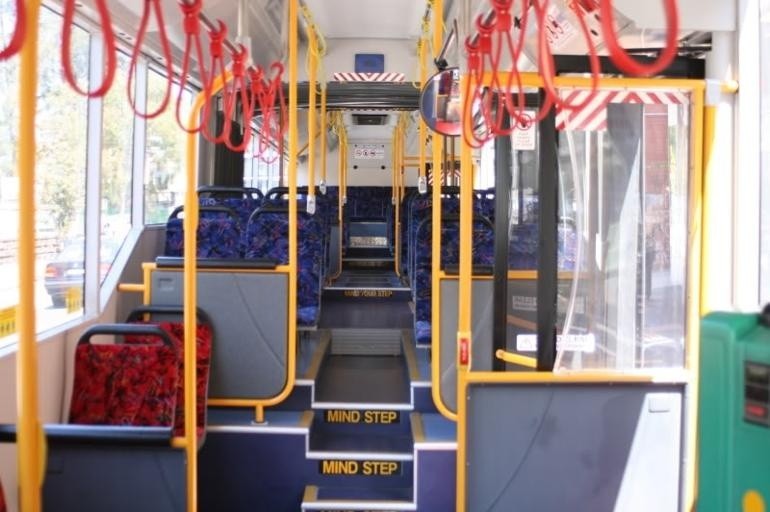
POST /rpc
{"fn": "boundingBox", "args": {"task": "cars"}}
[43,232,120,306]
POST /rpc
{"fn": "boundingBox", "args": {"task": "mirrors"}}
[419,68,495,136]
